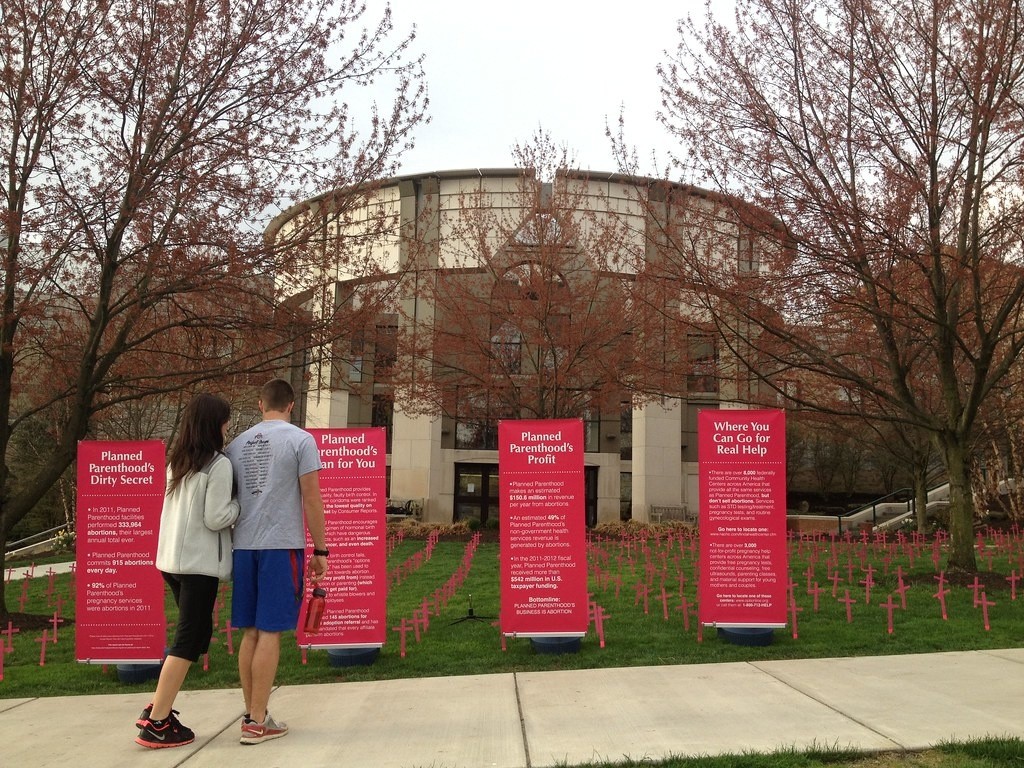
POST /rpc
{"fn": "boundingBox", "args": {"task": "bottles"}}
[304,581,327,635]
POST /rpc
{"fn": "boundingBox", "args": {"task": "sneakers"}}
[239,716,289,744]
[135,718,194,749]
[135,705,191,732]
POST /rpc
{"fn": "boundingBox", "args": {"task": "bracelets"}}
[313,548,330,556]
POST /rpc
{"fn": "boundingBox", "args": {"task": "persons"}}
[135,392,241,749]
[224,379,328,744]
